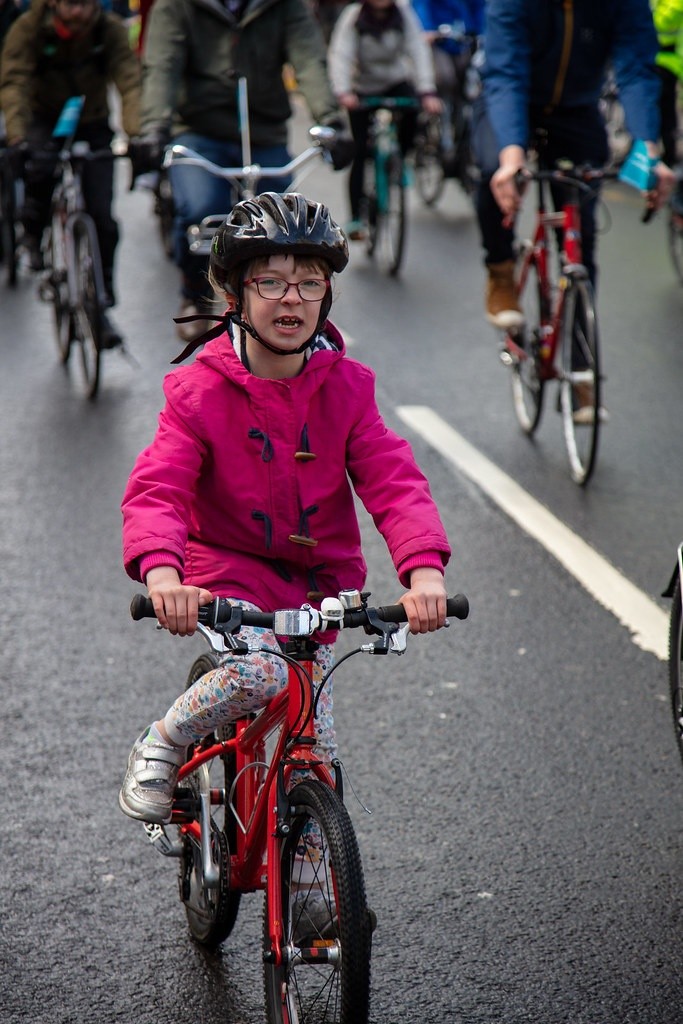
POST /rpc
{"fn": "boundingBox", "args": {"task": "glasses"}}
[243,277,331,302]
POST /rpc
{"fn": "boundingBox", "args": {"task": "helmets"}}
[209,193,350,294]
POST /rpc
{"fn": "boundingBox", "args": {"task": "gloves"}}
[127,129,171,173]
[322,135,355,170]
[9,140,40,175]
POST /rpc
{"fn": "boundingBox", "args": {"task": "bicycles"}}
[657,543,683,765]
[0,20,683,407]
[127,586,470,1024]
[497,161,662,485]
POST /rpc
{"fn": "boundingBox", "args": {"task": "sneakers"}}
[293,892,375,948]
[485,263,525,328]
[557,380,609,423]
[118,717,186,822]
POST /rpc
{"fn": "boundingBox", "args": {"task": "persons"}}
[121,190,451,948]
[0,1,681,426]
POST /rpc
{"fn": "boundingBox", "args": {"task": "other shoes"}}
[29,245,45,272]
[351,216,370,240]
[95,314,122,350]
[175,294,212,340]
